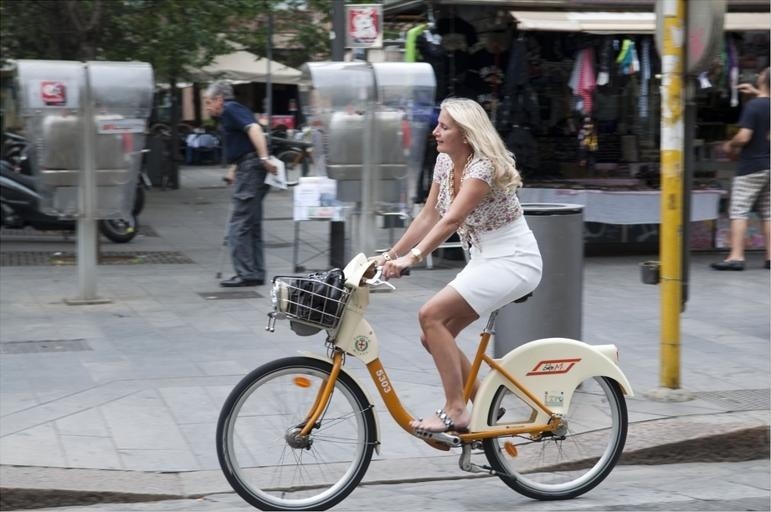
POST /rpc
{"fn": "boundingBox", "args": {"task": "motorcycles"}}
[0,159,141,243]
[1,130,154,217]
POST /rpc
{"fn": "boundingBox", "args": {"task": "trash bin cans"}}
[492,201,584,390]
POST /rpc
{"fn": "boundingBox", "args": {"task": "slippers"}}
[410,409,469,435]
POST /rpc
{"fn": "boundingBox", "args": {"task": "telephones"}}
[403,121,411,145]
[123,132,134,154]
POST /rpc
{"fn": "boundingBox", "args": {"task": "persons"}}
[368,96,544,431]
[713,67,771,270]
[205,81,278,287]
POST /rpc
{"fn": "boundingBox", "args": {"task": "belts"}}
[237,152,258,162]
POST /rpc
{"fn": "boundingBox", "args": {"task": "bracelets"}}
[381,252,392,261]
[259,157,272,162]
[391,248,400,259]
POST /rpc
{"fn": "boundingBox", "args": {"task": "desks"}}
[522,181,728,247]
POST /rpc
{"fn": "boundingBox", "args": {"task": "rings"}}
[390,272,395,275]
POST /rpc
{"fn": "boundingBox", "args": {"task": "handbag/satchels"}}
[288,268,347,327]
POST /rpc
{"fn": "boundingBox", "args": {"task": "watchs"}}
[410,248,424,264]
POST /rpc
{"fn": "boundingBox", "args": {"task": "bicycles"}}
[267,133,316,188]
[214,256,636,512]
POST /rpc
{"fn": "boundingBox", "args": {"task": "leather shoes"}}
[712,261,745,270]
[220,277,264,287]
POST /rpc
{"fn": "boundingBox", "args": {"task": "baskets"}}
[271,276,355,337]
